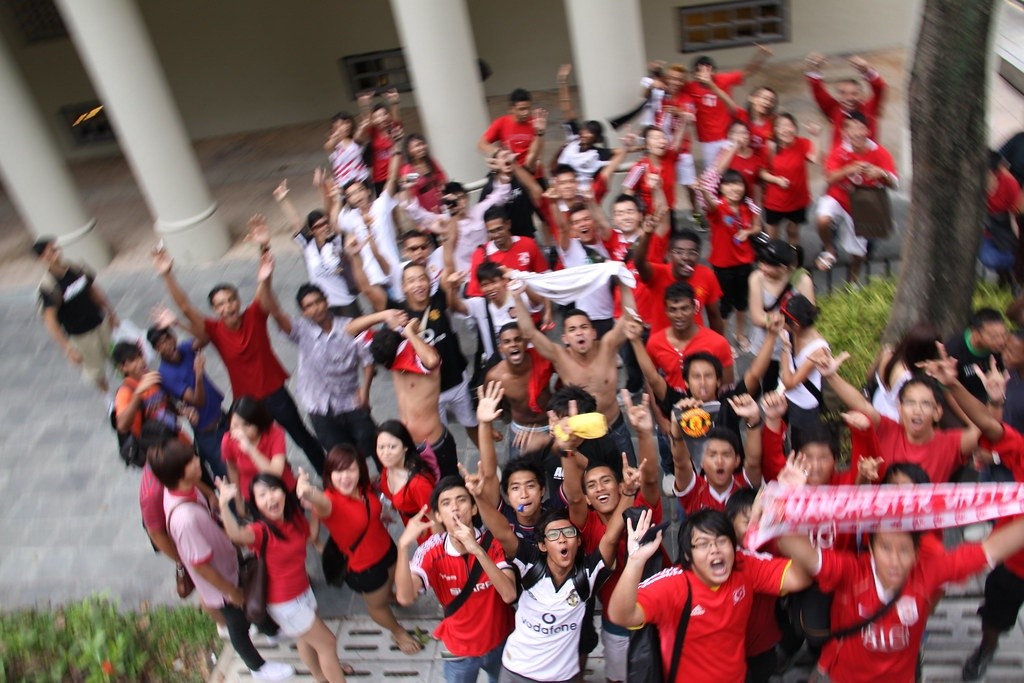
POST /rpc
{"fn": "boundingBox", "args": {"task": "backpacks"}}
[107,383,149,469]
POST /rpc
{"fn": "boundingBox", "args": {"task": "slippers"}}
[389,633,421,655]
[815,250,837,272]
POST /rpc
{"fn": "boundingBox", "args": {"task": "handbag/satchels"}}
[339,231,361,295]
[175,566,195,598]
[322,534,349,588]
[626,624,667,683]
[243,553,268,625]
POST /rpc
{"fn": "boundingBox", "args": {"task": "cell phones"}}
[637,520,671,544]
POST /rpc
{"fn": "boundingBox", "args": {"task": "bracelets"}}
[622,490,636,496]
[745,416,762,429]
[881,170,888,178]
[669,431,683,445]
[782,347,787,353]
[538,132,544,136]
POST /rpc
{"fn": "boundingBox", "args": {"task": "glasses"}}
[779,293,800,324]
[407,244,427,251]
[544,526,578,541]
[312,218,331,232]
[901,399,932,415]
[690,535,730,551]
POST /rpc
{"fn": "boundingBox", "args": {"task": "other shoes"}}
[963,641,1000,683]
[339,662,354,676]
[772,646,794,674]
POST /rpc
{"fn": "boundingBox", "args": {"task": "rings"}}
[803,470,808,476]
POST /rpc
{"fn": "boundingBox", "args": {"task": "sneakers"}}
[251,661,296,682]
[267,628,293,646]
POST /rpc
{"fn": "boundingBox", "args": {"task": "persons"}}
[111,45,1024,683]
[32,234,120,411]
